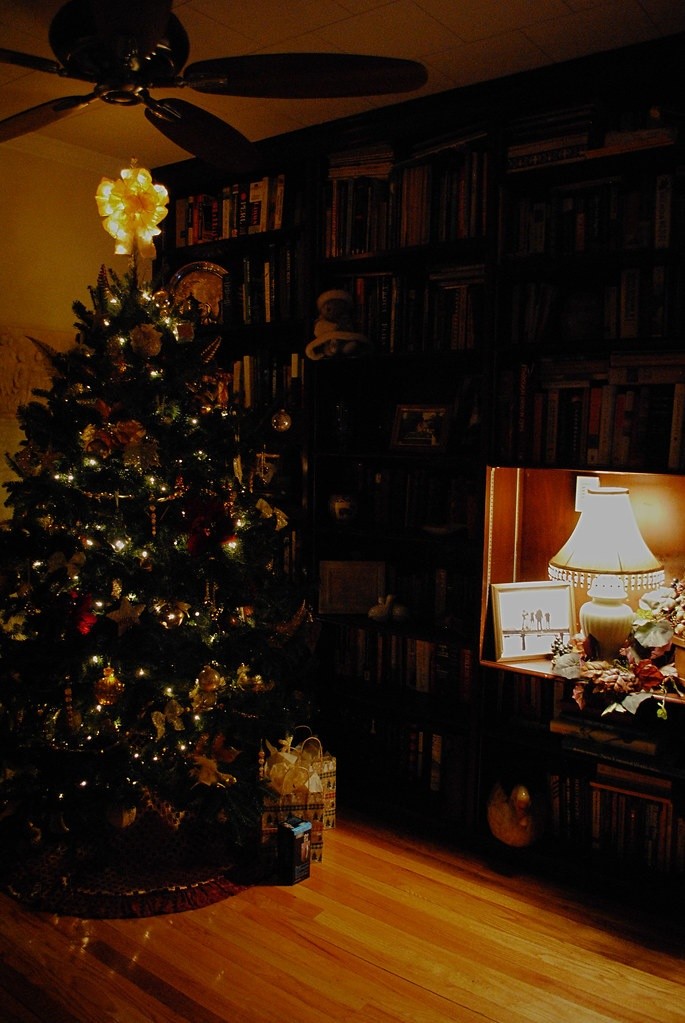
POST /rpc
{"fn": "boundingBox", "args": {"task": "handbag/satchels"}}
[291,726,336,830]
[261,767,323,864]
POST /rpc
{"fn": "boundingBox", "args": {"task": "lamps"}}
[547,487,666,662]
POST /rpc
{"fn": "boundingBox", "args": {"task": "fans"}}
[0,0,429,174]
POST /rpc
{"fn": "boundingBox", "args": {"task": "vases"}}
[672,632,685,687]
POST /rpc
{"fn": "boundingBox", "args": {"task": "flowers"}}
[550,572,685,719]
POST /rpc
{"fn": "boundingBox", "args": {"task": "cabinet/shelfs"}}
[151,28,685,959]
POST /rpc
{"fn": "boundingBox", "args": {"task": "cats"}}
[305,288,368,361]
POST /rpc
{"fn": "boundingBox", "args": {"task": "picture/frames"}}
[389,405,454,450]
[319,561,386,615]
[491,580,577,662]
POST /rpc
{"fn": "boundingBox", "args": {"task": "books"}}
[175,103,685,871]
